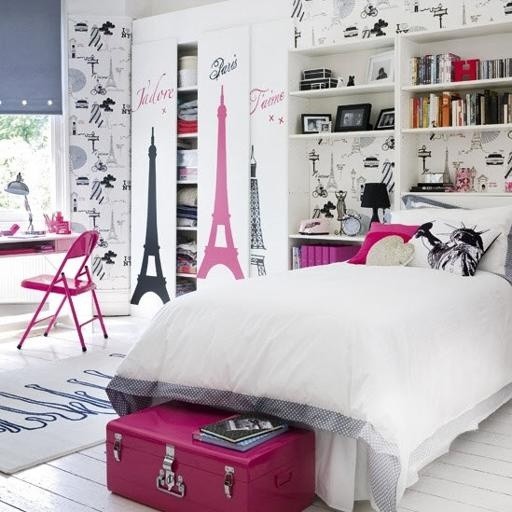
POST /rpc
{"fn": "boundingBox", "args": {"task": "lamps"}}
[360,182,390,230]
[4,173,44,235]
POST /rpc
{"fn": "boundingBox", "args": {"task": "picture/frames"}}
[364,48,398,84]
[301,102,394,134]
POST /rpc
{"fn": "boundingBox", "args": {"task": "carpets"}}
[0,349,131,478]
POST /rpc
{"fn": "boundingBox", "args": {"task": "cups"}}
[178,57,197,87]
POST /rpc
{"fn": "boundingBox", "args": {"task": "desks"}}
[1,223,95,327]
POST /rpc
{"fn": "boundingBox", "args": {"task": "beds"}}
[128,196,512,512]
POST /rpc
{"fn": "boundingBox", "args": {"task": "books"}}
[407,51,512,128]
[199,411,287,445]
[191,413,291,453]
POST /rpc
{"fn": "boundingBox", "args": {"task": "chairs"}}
[14,229,110,354]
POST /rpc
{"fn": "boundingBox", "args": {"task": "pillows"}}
[344,206,511,277]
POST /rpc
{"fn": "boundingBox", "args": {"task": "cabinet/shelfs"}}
[129,23,253,314]
[287,21,511,271]
